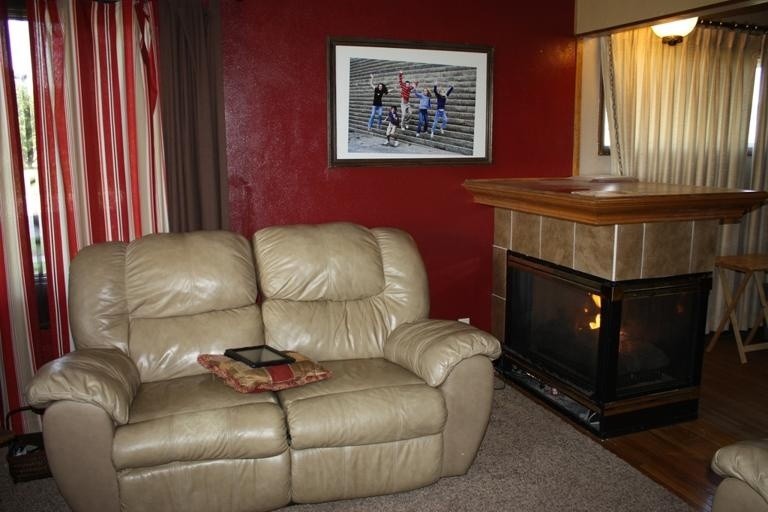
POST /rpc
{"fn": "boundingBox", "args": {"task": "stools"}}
[707,254,767,364]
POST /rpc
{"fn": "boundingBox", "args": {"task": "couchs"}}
[26,222,501,512]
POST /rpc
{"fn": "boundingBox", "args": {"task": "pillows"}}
[197,350,333,394]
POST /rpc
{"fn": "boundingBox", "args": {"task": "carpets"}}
[0,373,702,510]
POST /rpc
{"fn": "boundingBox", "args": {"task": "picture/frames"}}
[327,36,494,168]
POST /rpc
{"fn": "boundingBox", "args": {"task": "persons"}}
[382,105,400,148]
[410,83,434,138]
[398,69,419,131]
[367,72,395,132]
[428,79,456,139]
